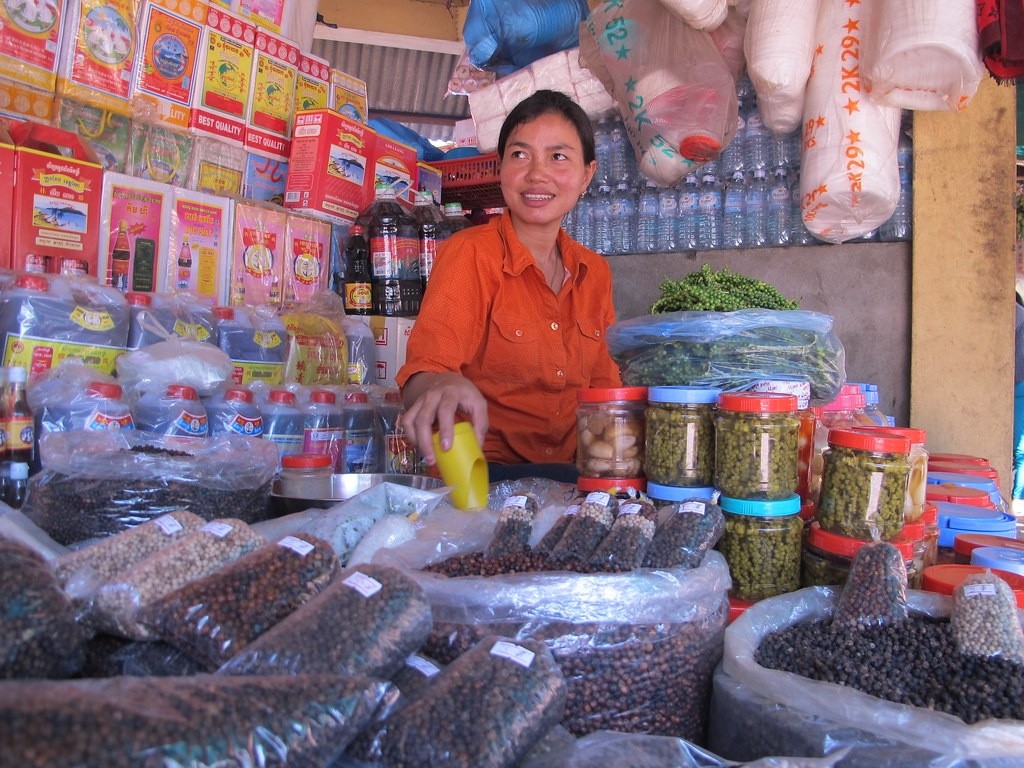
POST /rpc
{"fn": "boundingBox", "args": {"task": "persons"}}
[393,90,628,483]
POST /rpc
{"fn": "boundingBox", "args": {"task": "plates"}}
[801,0,977,242]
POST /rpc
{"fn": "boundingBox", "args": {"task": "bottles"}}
[0,184,476,509]
[560,105,911,253]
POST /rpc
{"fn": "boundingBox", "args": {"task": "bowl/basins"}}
[269,474,445,516]
[601,0,735,188]
[745,0,821,140]
[459,0,590,76]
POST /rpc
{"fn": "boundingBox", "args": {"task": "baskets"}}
[420,152,506,210]
